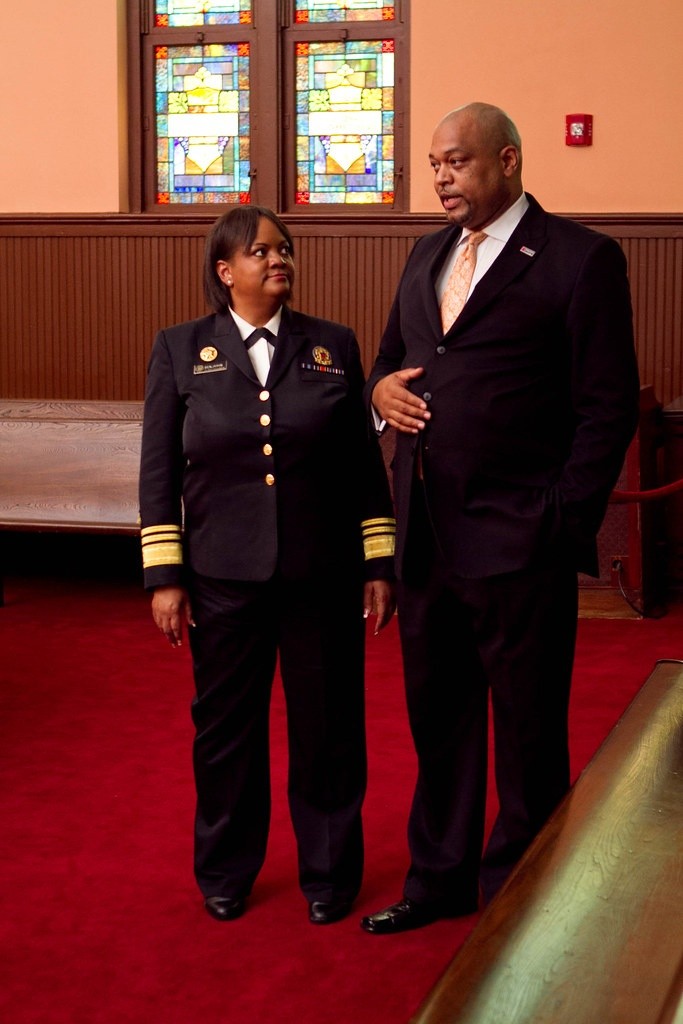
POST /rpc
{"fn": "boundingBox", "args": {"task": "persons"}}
[354,103,648,934]
[129,205,397,928]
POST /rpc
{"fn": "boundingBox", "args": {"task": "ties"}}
[439,231,487,333]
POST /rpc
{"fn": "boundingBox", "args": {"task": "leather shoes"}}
[360,900,439,934]
[203,893,248,919]
[307,901,352,922]
